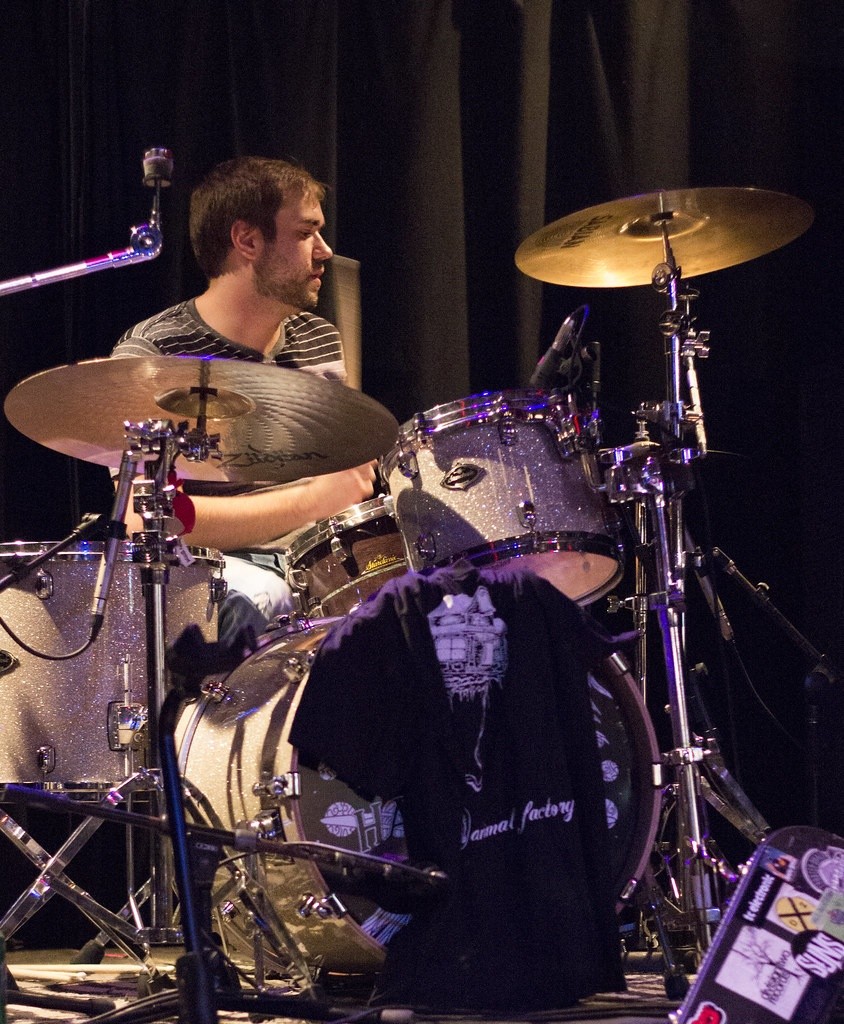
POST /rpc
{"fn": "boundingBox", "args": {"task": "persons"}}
[110,157,381,668]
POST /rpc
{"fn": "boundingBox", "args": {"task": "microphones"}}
[684,529,735,644]
[143,149,172,186]
[327,850,449,896]
[87,452,142,638]
[530,314,577,390]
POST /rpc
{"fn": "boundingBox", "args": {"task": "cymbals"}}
[3,355,401,484]
[597,442,742,465]
[513,185,816,290]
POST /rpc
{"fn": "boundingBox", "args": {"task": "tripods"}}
[0,420,331,1024]
[614,254,772,995]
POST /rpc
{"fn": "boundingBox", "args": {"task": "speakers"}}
[678,825,844,1024]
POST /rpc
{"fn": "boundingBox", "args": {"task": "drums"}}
[377,389,627,607]
[0,542,288,793]
[282,494,411,617]
[171,565,665,977]
